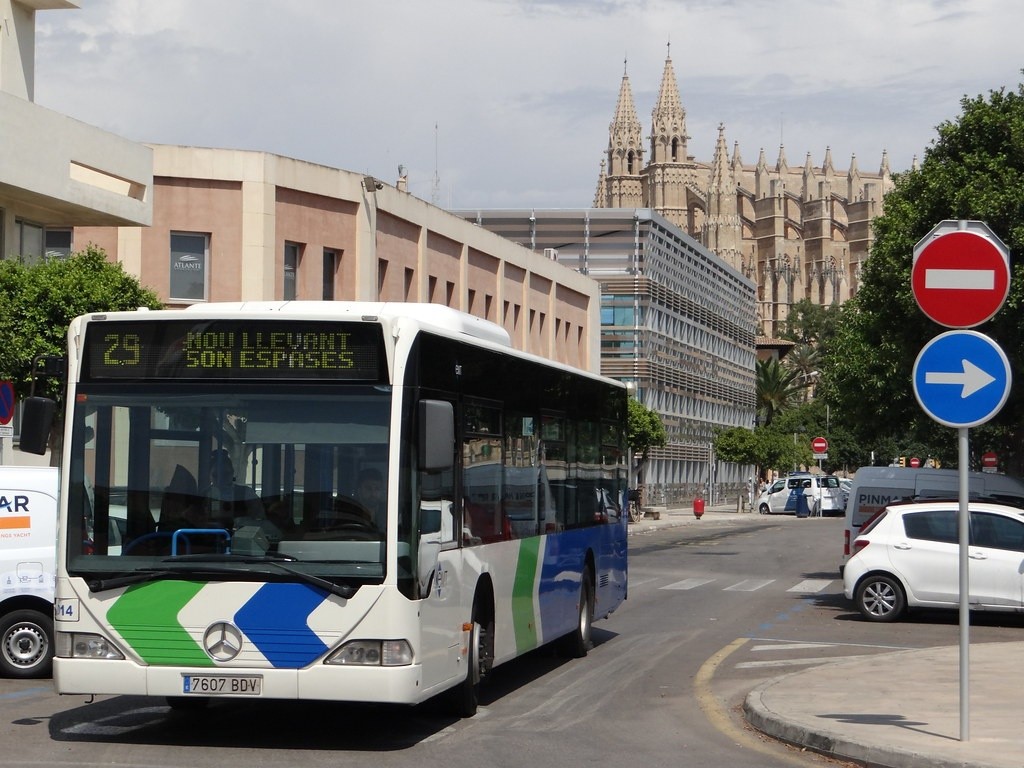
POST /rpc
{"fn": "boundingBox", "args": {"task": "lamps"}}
[361,177,384,192]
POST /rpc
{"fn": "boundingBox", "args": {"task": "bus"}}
[18,299,631,720]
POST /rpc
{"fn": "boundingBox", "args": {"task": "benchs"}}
[642,506,666,520]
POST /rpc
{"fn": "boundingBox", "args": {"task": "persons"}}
[333,468,386,535]
[183,450,266,523]
[747,477,771,505]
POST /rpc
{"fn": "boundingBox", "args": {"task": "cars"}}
[841,495,1024,623]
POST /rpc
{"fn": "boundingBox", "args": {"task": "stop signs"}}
[811,437,829,454]
[981,452,998,468]
[910,457,920,468]
[910,230,1012,330]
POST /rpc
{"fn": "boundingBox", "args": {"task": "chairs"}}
[790,482,797,488]
[424,462,628,540]
[803,482,811,488]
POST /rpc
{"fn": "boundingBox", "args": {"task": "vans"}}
[755,471,845,515]
[839,464,1024,580]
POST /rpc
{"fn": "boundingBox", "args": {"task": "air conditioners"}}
[544,248,559,261]
